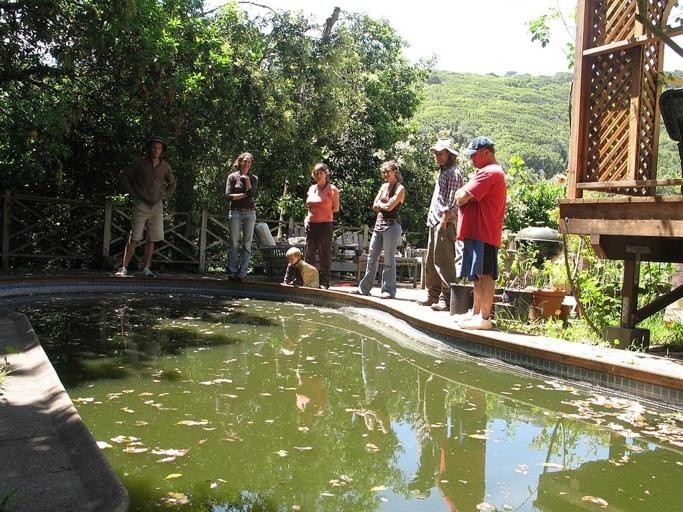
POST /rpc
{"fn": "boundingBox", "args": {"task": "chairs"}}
[659,87,683,195]
[252,222,306,280]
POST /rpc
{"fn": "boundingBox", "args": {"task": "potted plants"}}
[449,229,583,324]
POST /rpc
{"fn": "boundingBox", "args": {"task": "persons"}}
[302,161,339,290]
[113,135,177,278]
[416,138,463,312]
[121,293,485,512]
[282,247,320,287]
[452,135,507,331]
[357,159,405,300]
[224,152,259,282]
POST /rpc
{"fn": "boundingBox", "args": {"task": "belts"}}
[232,207,254,212]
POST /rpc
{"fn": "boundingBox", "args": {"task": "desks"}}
[353,255,418,288]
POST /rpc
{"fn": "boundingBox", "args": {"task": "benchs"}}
[279,224,428,290]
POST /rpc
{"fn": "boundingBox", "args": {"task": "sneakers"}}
[115,267,127,277]
[380,291,390,298]
[432,300,450,310]
[452,309,474,322]
[459,314,492,329]
[142,268,155,278]
[417,298,429,306]
[350,289,360,294]
[228,271,247,281]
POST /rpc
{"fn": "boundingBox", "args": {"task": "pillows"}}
[254,222,278,256]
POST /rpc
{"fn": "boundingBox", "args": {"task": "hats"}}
[465,137,494,155]
[146,136,167,152]
[430,138,458,156]
